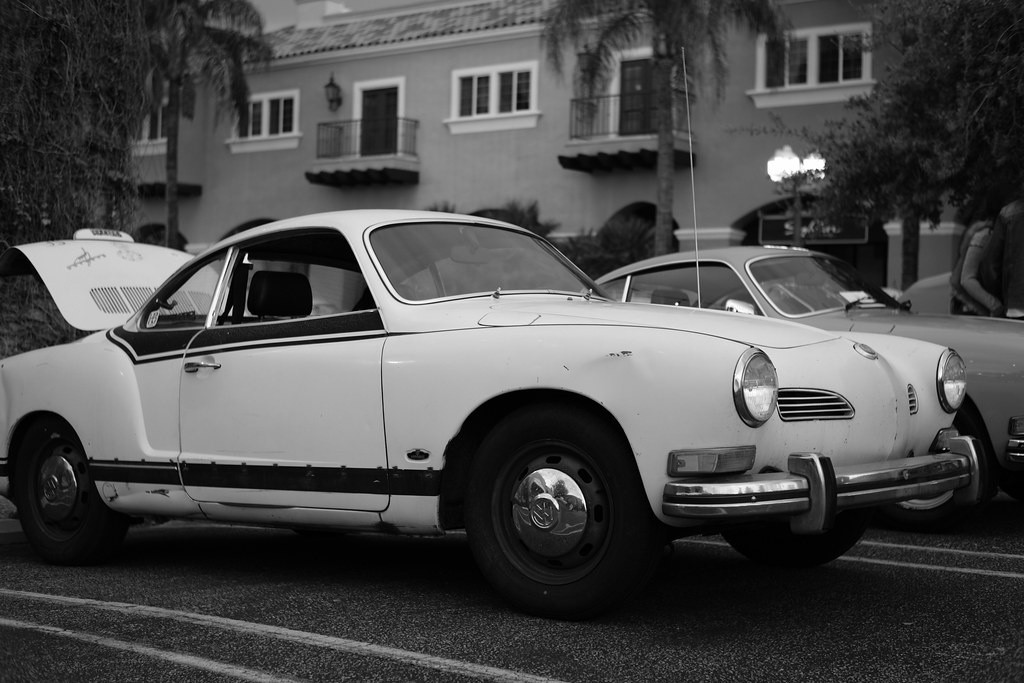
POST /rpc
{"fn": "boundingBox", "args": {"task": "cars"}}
[0,203,987,619]
[591,243,1024,531]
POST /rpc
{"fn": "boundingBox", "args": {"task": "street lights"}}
[765,146,828,249]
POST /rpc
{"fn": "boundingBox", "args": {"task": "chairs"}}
[651,289,691,307]
[248,269,314,322]
[358,284,415,308]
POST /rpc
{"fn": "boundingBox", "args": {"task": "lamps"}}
[323,71,342,112]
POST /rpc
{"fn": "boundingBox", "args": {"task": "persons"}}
[949,184,1003,320]
[992,192,1024,320]
